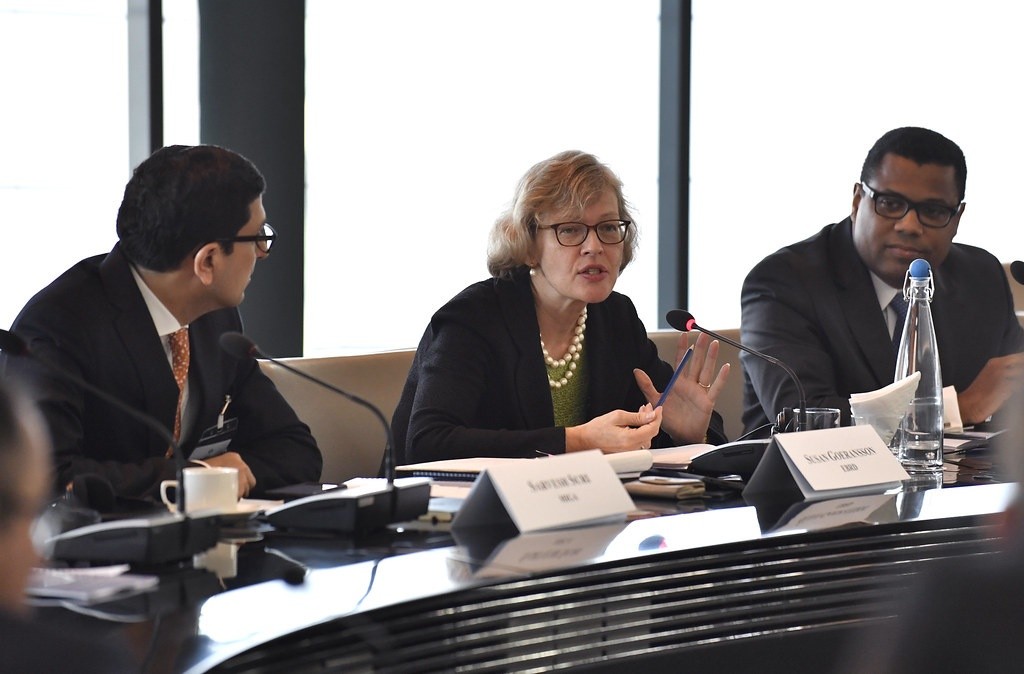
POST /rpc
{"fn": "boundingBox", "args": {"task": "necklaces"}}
[540,304,588,387]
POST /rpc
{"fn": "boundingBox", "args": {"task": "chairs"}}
[254,348,419,492]
[648,326,743,443]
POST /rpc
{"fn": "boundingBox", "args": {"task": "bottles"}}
[888,259,944,472]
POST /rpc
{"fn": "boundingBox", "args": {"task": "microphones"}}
[1010,260,1024,286]
[1,328,223,569]
[664,308,804,477]
[219,330,430,534]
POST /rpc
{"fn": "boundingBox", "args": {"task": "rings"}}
[641,445,645,449]
[698,381,711,387]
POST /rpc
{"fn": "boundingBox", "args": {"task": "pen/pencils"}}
[653,344,695,410]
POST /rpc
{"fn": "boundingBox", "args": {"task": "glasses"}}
[192,223,279,259]
[862,180,961,228]
[0,495,63,561]
[532,220,631,247]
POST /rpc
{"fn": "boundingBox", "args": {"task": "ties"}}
[889,292,911,359]
[165,325,190,459]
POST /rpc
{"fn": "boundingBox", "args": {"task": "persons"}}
[376,150,731,480]
[738,127,1024,440]
[0,144,323,615]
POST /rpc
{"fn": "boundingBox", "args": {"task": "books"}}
[394,443,715,531]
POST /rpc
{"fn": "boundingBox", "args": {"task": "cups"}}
[792,407,840,432]
[850,414,905,467]
[160,467,239,516]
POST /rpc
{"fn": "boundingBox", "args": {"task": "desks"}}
[42,435,1024,673]
